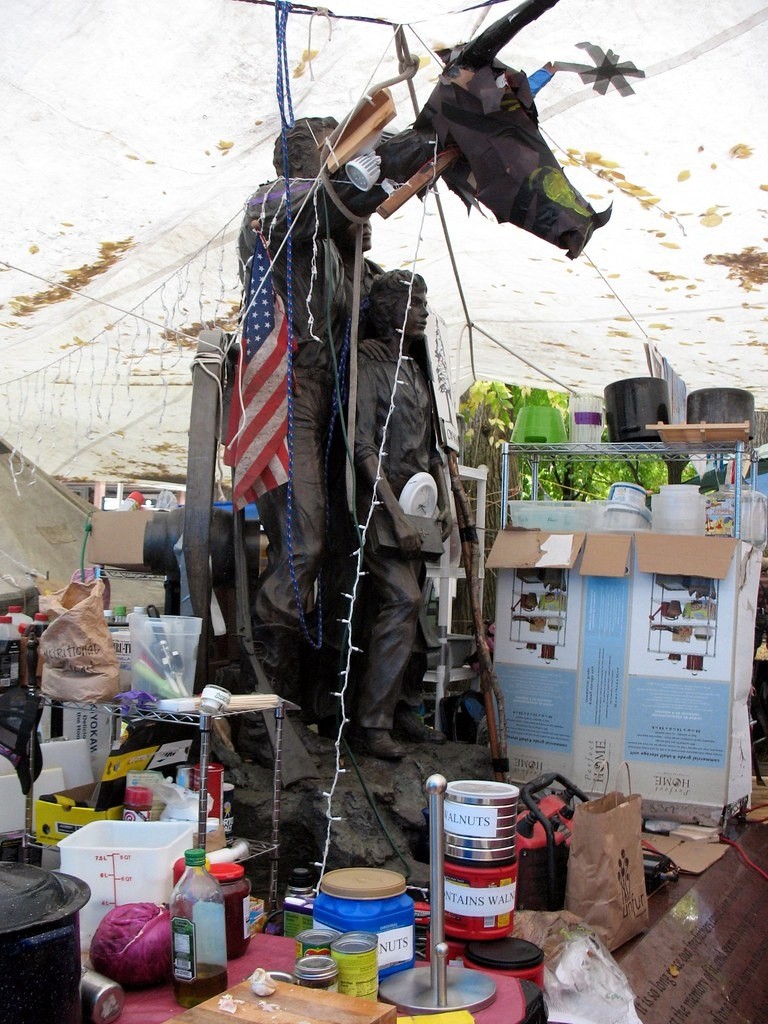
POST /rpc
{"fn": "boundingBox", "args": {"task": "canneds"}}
[208,862,252,961]
[265,867,416,1002]
[443,780,544,988]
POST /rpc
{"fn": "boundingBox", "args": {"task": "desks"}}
[162,978,397,1024]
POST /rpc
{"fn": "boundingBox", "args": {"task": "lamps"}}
[343,150,384,192]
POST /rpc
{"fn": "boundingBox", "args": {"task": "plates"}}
[398,472,438,518]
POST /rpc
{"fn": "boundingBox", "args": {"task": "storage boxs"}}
[57,818,194,951]
[36,775,129,848]
[89,509,156,570]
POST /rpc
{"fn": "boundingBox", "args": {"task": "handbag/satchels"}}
[563,760,651,953]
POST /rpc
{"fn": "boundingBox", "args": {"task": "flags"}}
[222,234,297,513]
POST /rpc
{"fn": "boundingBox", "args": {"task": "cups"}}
[568,395,606,443]
[223,782,235,840]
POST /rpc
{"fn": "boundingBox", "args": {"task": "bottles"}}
[0,605,52,691]
[648,484,708,535]
[101,605,146,624]
[286,867,315,900]
[707,483,768,546]
[117,491,144,511]
[312,867,416,980]
[207,863,251,959]
[293,954,340,992]
[167,848,229,1008]
[462,936,547,993]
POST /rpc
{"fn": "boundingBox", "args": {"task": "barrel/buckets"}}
[128,614,203,699]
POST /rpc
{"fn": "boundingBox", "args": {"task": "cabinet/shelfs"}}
[26,685,286,927]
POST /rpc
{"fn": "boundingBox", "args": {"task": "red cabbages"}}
[89,903,173,988]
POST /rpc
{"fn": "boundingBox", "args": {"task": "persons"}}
[237,99,453,764]
